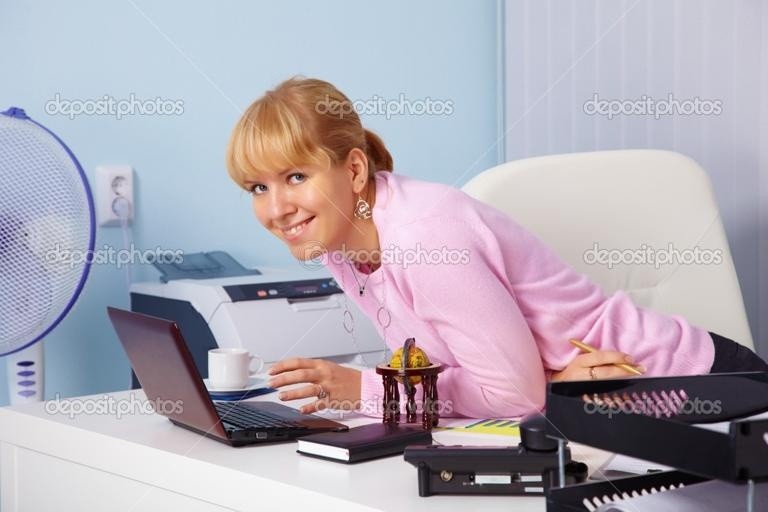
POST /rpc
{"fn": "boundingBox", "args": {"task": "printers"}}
[130,251,394,391]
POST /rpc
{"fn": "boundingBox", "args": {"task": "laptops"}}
[105,305,349,447]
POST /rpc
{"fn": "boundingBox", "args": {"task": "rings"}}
[589,369,598,380]
[317,384,326,399]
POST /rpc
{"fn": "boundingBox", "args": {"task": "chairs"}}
[455,147,755,384]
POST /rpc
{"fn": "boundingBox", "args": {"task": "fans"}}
[1,106,95,405]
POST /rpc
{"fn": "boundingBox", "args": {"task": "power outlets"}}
[96,165,135,229]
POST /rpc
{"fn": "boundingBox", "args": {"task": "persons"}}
[224,77,768,422]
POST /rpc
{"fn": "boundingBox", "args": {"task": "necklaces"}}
[346,248,379,298]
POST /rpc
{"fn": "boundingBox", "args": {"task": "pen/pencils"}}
[570,338,645,376]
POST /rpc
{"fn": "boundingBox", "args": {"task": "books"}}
[432,417,678,478]
[296,422,433,466]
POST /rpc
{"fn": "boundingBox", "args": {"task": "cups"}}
[207,348,264,389]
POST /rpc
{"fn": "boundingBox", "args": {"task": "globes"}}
[377,338,444,429]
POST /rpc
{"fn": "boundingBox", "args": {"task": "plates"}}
[202,378,268,397]
